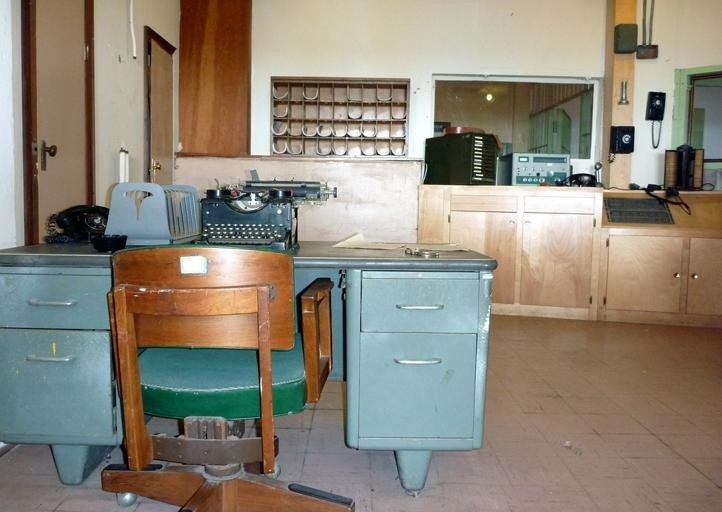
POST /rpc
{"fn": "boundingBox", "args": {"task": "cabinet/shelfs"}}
[449,195,595,309]
[269,76,411,157]
[345,269,494,451]
[1,266,125,446]
[171,152,424,244]
[606,235,722,328]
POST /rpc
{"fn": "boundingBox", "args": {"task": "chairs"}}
[107,245,335,512]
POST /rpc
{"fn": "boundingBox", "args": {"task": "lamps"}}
[485,89,493,101]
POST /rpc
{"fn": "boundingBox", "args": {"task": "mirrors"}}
[434,80,594,159]
[687,72,722,163]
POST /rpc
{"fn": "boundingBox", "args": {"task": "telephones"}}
[568,173,598,187]
[609,125,636,155]
[56,204,111,243]
[645,91,666,122]
